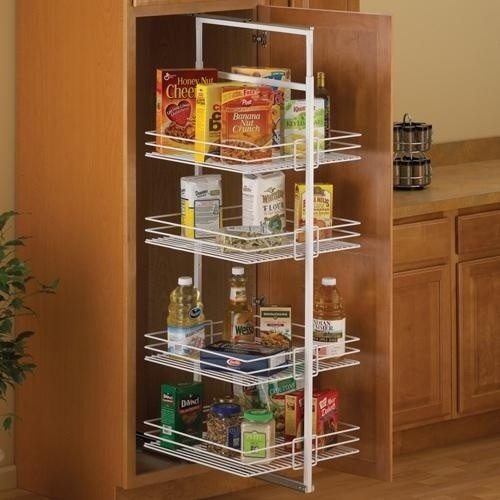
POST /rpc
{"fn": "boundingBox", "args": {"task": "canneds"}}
[210,394,241,416]
[240,409,276,465]
[206,402,241,456]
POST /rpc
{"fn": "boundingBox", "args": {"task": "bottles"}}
[395,123,432,185]
[225,267,254,342]
[315,277,345,361]
[167,276,204,361]
[315,72,330,151]
[207,404,276,465]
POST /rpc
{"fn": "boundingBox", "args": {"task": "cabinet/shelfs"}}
[15,0,391,496]
[389,208,500,432]
